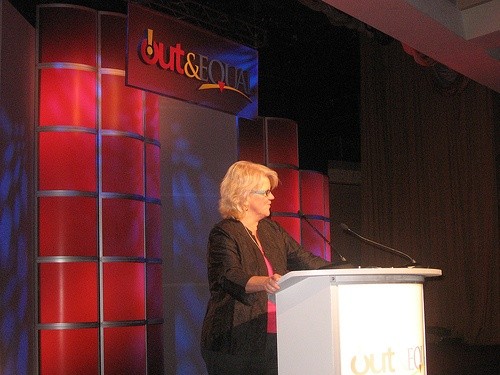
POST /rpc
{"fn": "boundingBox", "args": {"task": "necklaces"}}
[231,216,264,254]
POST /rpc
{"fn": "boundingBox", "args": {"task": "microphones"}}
[339,223,426,268]
[298,209,352,268]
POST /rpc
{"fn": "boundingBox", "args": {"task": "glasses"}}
[251,190,271,197]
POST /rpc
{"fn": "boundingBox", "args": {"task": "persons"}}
[201,161,332,375]
